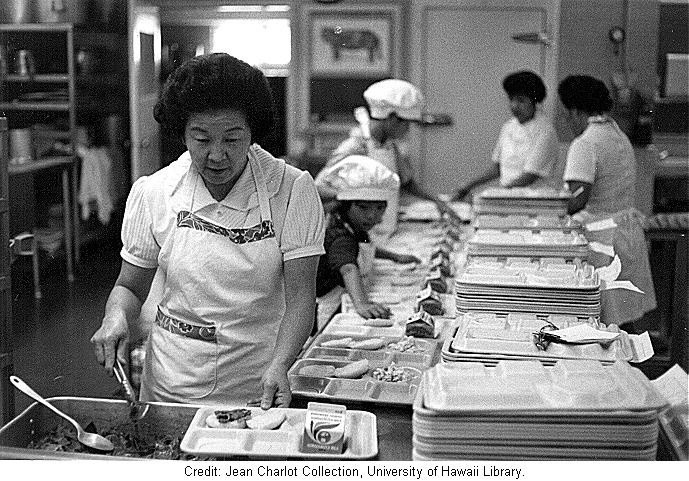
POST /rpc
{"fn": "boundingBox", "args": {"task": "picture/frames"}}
[310,14,393,76]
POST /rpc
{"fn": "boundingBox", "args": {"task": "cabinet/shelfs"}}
[640,158,689,382]
[0,24,131,259]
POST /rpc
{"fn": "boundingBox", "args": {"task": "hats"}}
[556,71,614,115]
[362,77,433,124]
[314,153,401,203]
[152,52,278,138]
[502,70,546,104]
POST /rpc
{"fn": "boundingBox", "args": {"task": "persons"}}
[307,154,422,342]
[560,74,659,364]
[313,78,462,224]
[446,70,558,204]
[91,52,326,410]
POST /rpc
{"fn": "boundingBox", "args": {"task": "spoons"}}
[9,374,117,453]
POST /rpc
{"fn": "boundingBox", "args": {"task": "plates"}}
[466,227,592,263]
[471,186,573,221]
[315,313,448,340]
[438,310,634,367]
[341,292,458,322]
[409,359,668,461]
[453,259,603,326]
[286,358,429,408]
[179,405,378,460]
[300,333,438,369]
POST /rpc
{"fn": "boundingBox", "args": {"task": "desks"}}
[0,218,689,461]
[7,158,75,299]
